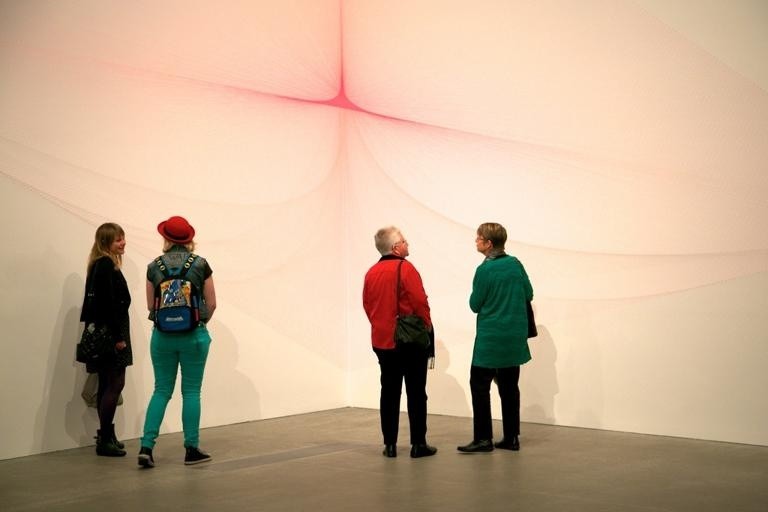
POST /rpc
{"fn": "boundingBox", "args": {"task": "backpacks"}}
[149,255,200,334]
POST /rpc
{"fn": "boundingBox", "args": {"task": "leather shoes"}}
[383,445,396,457]
[410,445,437,458]
[457,438,494,453]
[497,438,520,450]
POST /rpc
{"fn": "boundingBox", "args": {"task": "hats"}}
[156,216,194,245]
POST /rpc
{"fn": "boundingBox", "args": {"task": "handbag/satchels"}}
[526,302,538,340]
[396,317,430,352]
[80,374,123,409]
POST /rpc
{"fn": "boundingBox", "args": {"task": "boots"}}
[93,430,126,457]
[102,423,125,447]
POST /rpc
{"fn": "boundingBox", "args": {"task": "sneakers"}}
[138,448,155,467]
[184,448,213,465]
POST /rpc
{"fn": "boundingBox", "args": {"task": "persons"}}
[362,225,437,459]
[78,222,131,459]
[138,215,217,468]
[456,222,533,453]
